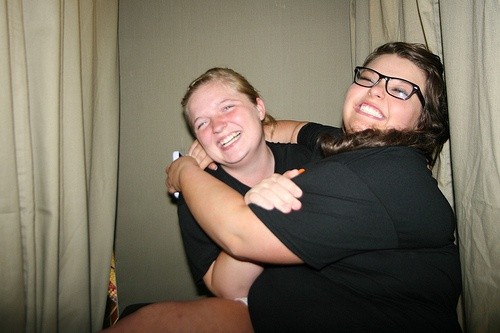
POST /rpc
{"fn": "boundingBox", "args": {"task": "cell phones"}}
[173,151,183,198]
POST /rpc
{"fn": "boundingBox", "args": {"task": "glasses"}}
[354,66,426,109]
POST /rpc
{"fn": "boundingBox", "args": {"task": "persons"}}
[96,42,463,333]
[177,68,320,299]
